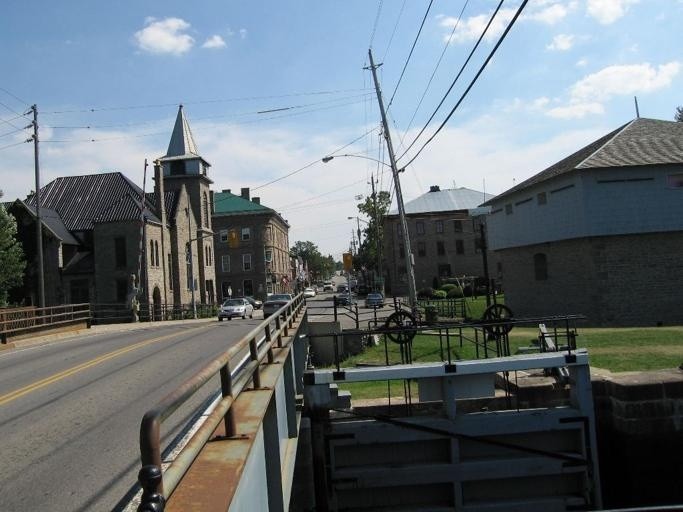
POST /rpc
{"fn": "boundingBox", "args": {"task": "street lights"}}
[321,154,420,321]
[348,216,386,298]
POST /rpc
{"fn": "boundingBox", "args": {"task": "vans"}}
[322,281,333,292]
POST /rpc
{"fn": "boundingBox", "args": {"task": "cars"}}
[263,294,293,320]
[240,296,263,310]
[216,297,254,322]
[365,293,384,308]
[337,284,346,294]
[303,287,316,298]
[335,293,354,306]
